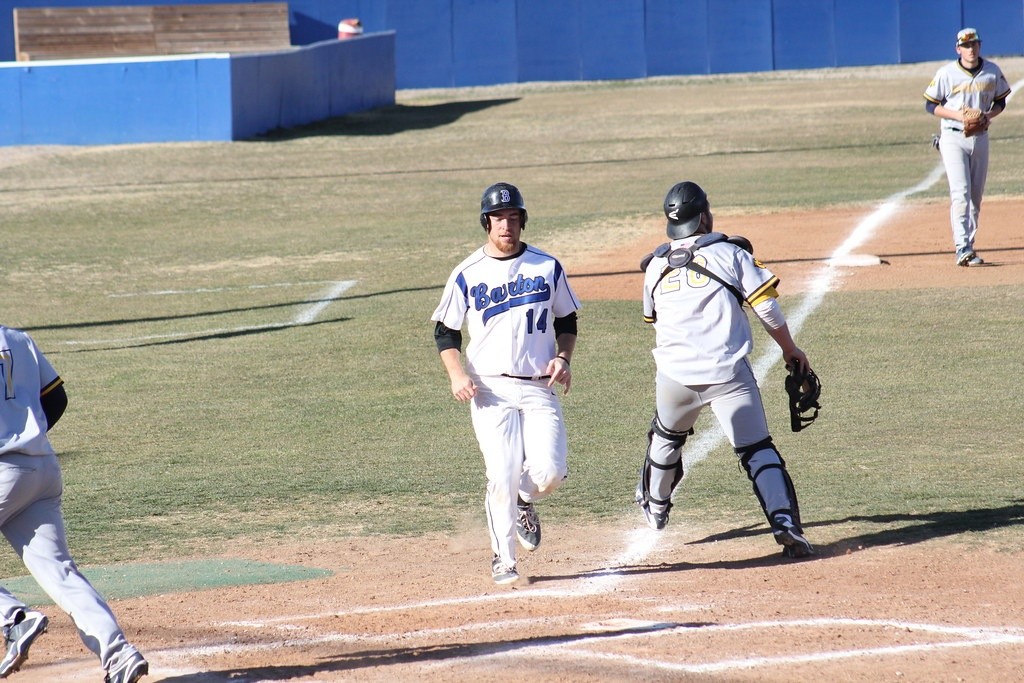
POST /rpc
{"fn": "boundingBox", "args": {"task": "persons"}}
[635,182,823,559]
[0,326,149,683]
[429,184,580,584]
[922,27,1012,266]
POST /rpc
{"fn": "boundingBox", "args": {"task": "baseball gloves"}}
[961,103,989,138]
[784,357,822,433]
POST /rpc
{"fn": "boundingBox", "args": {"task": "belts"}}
[504,375,552,381]
[952,128,959,132]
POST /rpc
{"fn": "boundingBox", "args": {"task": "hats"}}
[956,28,982,46]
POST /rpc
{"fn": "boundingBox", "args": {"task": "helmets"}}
[664,181,707,240]
[480,182,527,231]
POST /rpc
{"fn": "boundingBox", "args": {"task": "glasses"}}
[959,34,977,42]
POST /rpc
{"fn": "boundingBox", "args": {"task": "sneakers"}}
[492,556,519,585]
[956,249,975,266]
[636,479,667,529]
[773,516,815,559]
[516,500,541,552]
[0,609,48,678]
[967,256,984,265]
[107,653,150,683]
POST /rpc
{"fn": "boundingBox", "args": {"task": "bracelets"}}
[557,356,569,365]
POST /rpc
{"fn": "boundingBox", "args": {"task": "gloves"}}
[931,133,941,150]
